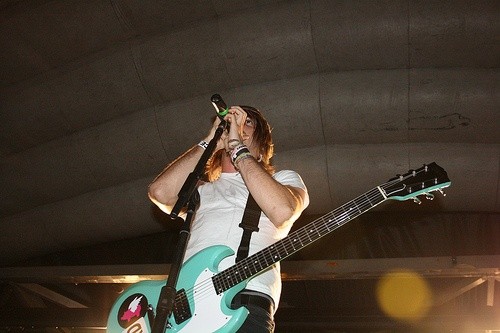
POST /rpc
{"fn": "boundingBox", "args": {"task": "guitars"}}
[106,162,451,333]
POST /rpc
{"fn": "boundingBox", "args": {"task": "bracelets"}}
[196,140,210,150]
[224,139,256,171]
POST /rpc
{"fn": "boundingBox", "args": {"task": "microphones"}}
[211,94,231,133]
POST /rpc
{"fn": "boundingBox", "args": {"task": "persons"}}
[146,106,310,333]
